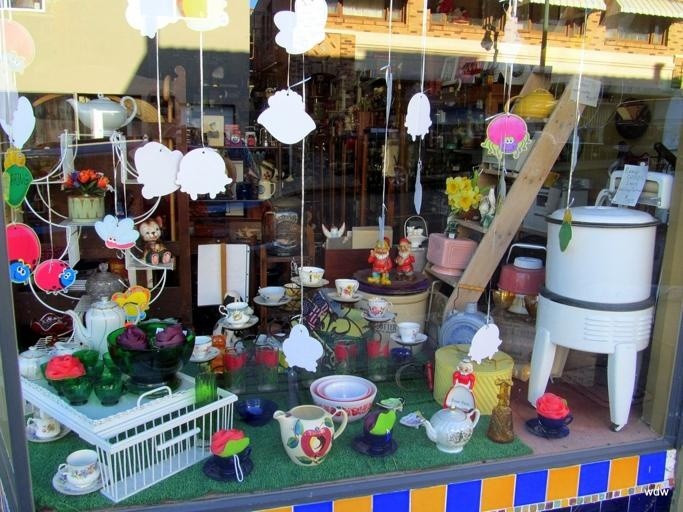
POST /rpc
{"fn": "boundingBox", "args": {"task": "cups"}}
[335,333,389,381]
[213,447,252,468]
[258,181,276,200]
[19,347,122,406]
[27,413,100,489]
[224,346,279,394]
[538,413,573,431]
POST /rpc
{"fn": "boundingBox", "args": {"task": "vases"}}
[68,195,106,224]
[457,206,481,221]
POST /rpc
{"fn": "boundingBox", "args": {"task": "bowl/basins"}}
[309,375,377,422]
[106,322,196,398]
[298,266,325,284]
[236,398,279,426]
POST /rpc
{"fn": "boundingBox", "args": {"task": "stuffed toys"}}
[134,216,172,266]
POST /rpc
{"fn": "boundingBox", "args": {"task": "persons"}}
[393,237,418,283]
[367,240,395,287]
[443,359,478,421]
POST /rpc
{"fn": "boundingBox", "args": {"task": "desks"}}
[19,335,683,511]
[324,237,427,288]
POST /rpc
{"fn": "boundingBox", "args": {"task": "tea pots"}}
[273,405,348,468]
[416,403,481,454]
[63,296,141,358]
[66,93,138,138]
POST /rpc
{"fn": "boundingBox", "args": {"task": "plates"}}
[203,457,253,482]
[526,417,570,439]
[53,462,111,496]
[351,433,398,457]
[188,276,428,362]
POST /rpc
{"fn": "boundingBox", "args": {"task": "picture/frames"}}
[189,103,235,146]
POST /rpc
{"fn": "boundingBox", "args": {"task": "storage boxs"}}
[351,226,394,250]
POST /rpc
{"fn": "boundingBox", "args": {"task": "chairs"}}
[258,197,314,333]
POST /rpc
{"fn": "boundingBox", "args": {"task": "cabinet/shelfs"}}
[1,62,193,356]
[186,142,284,334]
[407,83,557,218]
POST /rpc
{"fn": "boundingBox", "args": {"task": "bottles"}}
[196,364,219,440]
[225,125,257,147]
[237,183,252,199]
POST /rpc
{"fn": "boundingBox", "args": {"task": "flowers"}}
[155,325,186,349]
[444,165,491,216]
[116,325,147,352]
[59,168,114,197]
[44,354,87,380]
[210,428,251,458]
[535,391,571,421]
